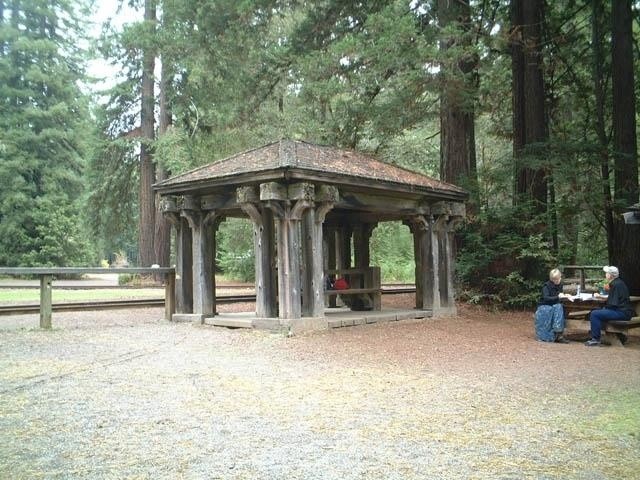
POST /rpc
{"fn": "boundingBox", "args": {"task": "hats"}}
[601,265,619,277]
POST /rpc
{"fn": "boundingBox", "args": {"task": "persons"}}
[584,266,632,345]
[533,267,569,344]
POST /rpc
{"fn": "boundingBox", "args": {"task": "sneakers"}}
[555,334,628,346]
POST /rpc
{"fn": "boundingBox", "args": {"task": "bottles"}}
[598,280,604,294]
[576,282,580,297]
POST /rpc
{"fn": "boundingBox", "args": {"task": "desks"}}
[561,294,640,347]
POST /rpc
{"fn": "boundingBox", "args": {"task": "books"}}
[580,293,609,302]
[559,292,574,303]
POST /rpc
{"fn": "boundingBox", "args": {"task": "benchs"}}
[565,313,589,320]
[606,316,640,333]
[324,288,379,311]
